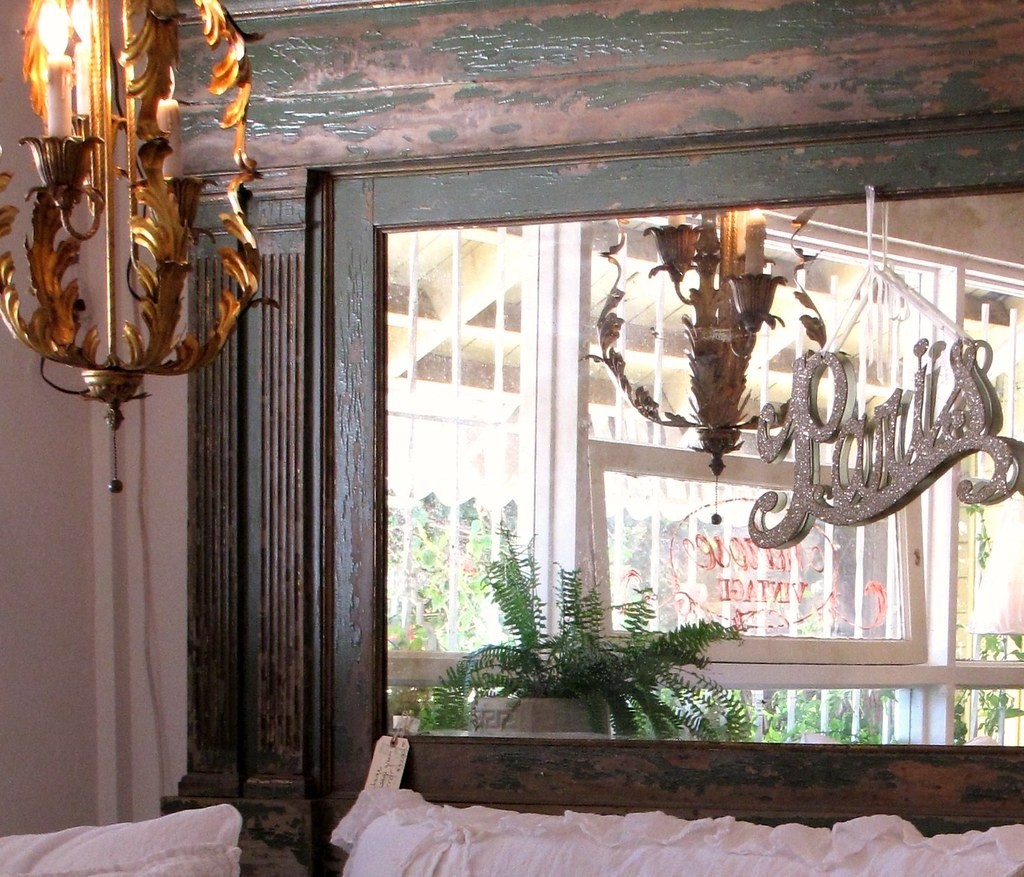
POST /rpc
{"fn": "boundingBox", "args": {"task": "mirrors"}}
[373,182,1023,758]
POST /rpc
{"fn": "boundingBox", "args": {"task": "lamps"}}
[2,0,266,495]
[578,207,829,531]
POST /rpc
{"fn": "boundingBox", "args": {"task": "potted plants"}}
[425,520,758,744]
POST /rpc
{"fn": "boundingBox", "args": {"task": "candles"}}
[43,52,73,141]
[74,40,94,117]
[744,219,766,275]
[154,98,182,182]
[668,215,687,227]
